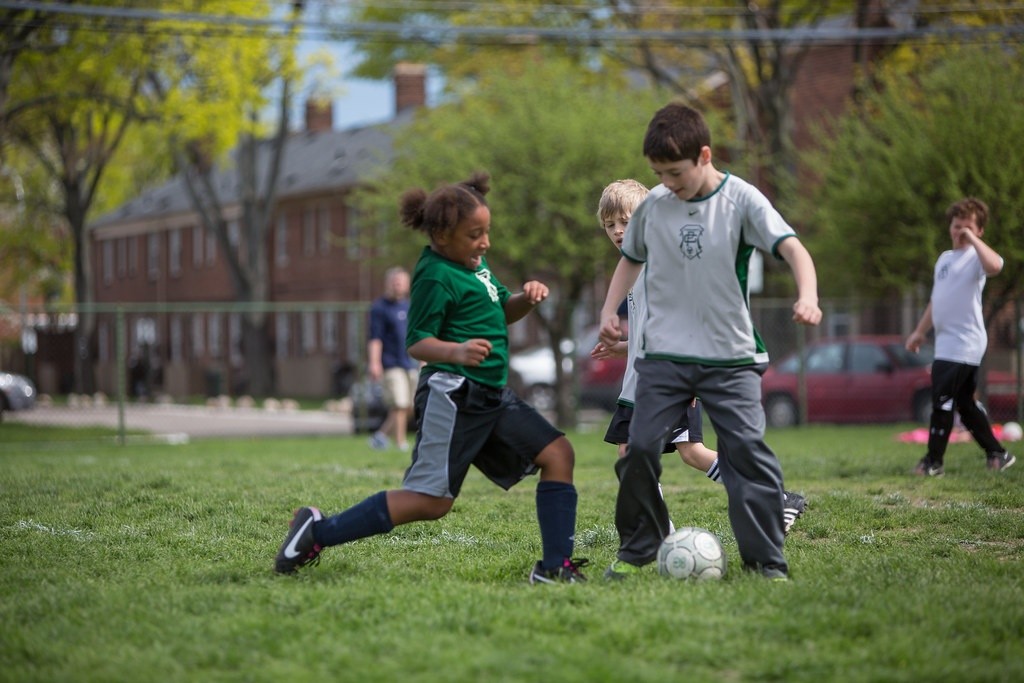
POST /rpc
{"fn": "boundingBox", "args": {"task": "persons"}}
[125,344,149,398]
[599,104,822,580]
[328,357,351,396]
[366,267,419,452]
[904,198,1016,475]
[591,179,806,533]
[275,172,586,584]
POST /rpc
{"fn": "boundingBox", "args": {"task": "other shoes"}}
[364,433,389,452]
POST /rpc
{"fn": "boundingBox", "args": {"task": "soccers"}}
[654,527,727,584]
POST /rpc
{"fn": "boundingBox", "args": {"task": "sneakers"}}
[779,490,807,541]
[984,448,1017,475]
[529,558,590,590]
[273,506,326,578]
[912,456,946,481]
[599,557,643,584]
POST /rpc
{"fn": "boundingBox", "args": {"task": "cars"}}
[348,321,628,433]
[0,369,38,417]
[758,336,1022,430]
